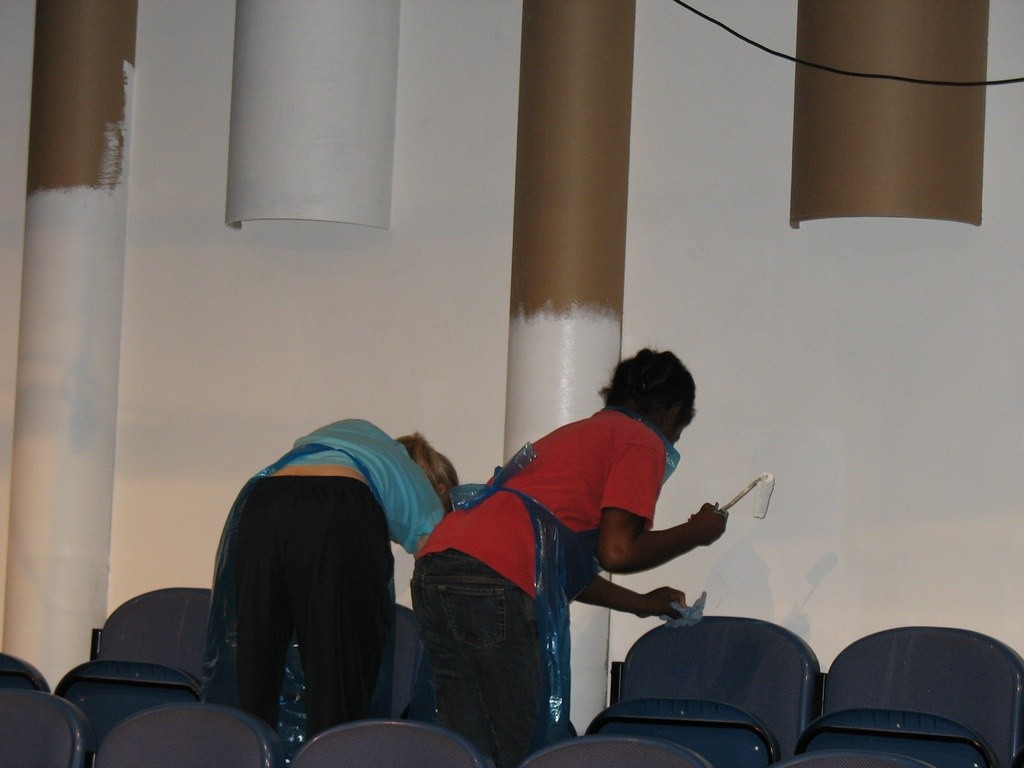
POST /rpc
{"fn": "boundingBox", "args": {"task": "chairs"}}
[0,583,1023,768]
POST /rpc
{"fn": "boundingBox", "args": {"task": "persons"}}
[207,418,459,759]
[409,348,729,768]
